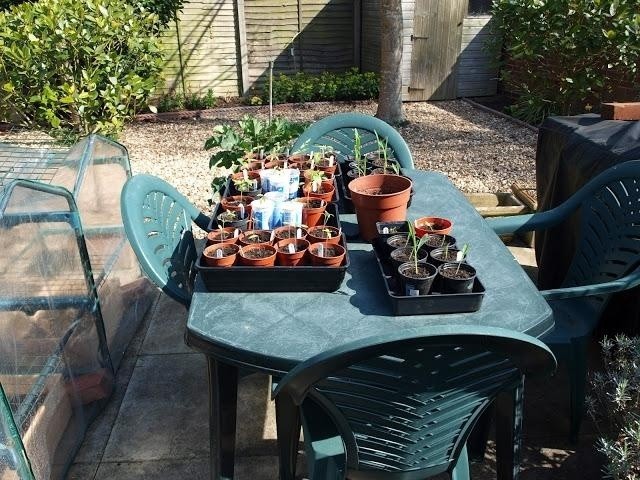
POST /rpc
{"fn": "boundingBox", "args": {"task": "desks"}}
[182,169,556,480]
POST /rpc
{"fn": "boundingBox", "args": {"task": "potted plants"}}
[195,113,348,268]
[342,126,414,210]
[374,215,478,296]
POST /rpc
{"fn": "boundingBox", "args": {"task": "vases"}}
[347,174,412,242]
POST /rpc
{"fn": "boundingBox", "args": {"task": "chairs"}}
[485,159,640,445]
[290,115,419,170]
[269,321,562,479]
[122,172,211,310]
